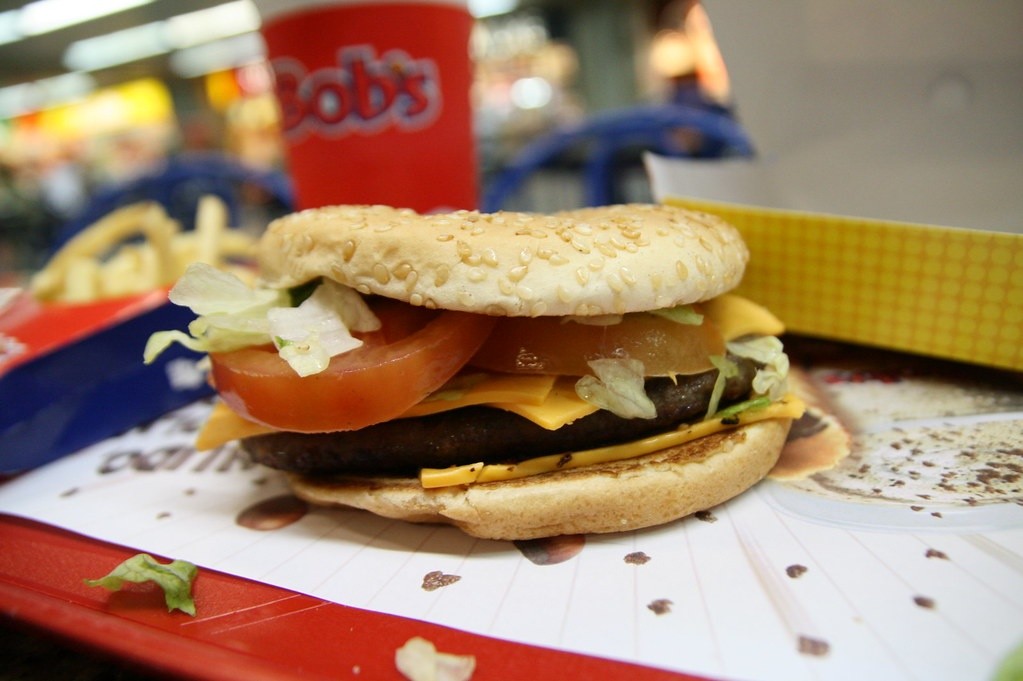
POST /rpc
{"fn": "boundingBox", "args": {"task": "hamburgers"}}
[140,204,807,542]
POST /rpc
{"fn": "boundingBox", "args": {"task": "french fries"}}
[25,193,249,305]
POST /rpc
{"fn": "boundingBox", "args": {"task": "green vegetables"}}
[84,554,198,618]
